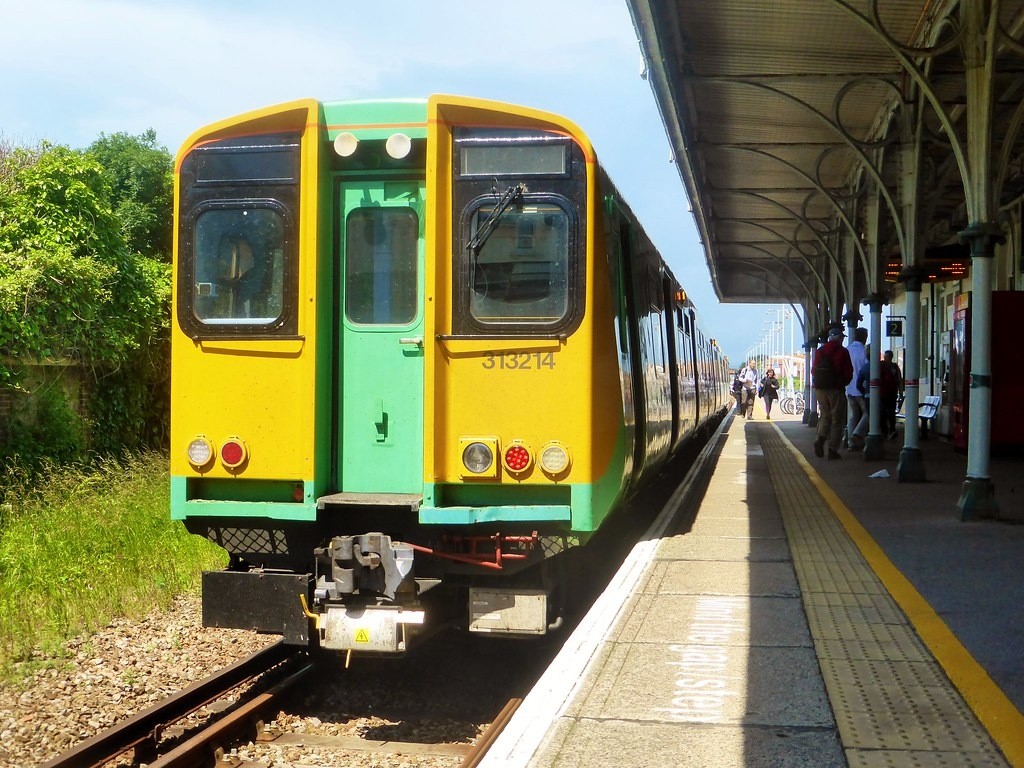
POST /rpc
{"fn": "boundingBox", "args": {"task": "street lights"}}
[744,304,797,416]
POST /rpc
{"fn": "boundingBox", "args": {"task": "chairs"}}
[895,393,941,440]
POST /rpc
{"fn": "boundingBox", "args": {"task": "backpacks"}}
[815,345,842,389]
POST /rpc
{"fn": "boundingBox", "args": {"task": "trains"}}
[170,93,729,682]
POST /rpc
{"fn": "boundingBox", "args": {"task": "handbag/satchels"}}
[732,376,743,392]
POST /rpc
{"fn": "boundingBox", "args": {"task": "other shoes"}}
[848,434,865,451]
[741,412,745,418]
[747,415,754,420]
[881,430,899,440]
[766,415,770,419]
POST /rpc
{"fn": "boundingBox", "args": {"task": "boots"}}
[829,447,842,460]
[814,436,827,458]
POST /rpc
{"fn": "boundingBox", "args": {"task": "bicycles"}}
[780,390,805,415]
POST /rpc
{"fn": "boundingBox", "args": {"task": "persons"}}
[732,360,758,420]
[811,328,854,460]
[760,368,780,420]
[845,327,903,451]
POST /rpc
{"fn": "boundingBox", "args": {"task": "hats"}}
[829,328,848,337]
